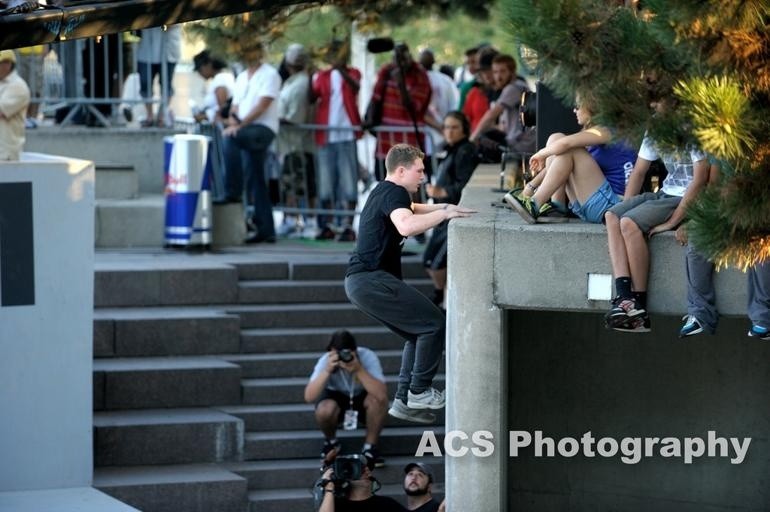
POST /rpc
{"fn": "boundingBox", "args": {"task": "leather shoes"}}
[212,194,243,206]
[244,231,275,244]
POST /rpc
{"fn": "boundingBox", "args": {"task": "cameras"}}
[337,350,352,364]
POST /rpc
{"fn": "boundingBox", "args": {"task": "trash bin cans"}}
[163,133,216,251]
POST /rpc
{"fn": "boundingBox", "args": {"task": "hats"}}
[0,49,16,63]
[404,462,436,483]
[284,42,307,67]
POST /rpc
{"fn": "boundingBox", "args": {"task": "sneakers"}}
[407,387,447,410]
[428,291,444,308]
[747,322,770,341]
[503,192,540,226]
[318,439,342,458]
[276,222,299,238]
[679,312,704,337]
[388,397,438,423]
[361,447,385,467]
[538,201,571,223]
[300,225,321,239]
[604,297,646,324]
[316,228,335,241]
[336,229,356,242]
[605,314,652,333]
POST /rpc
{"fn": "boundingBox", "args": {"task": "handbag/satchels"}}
[364,99,383,127]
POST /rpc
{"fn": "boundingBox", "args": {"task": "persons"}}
[501,84,638,224]
[304,330,387,471]
[675,157,769,342]
[602,83,708,333]
[316,457,408,512]
[469,53,529,164]
[0,9,499,303]
[403,461,445,512]
[344,144,445,423]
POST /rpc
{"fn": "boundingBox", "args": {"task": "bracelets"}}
[323,489,336,494]
[526,182,536,191]
